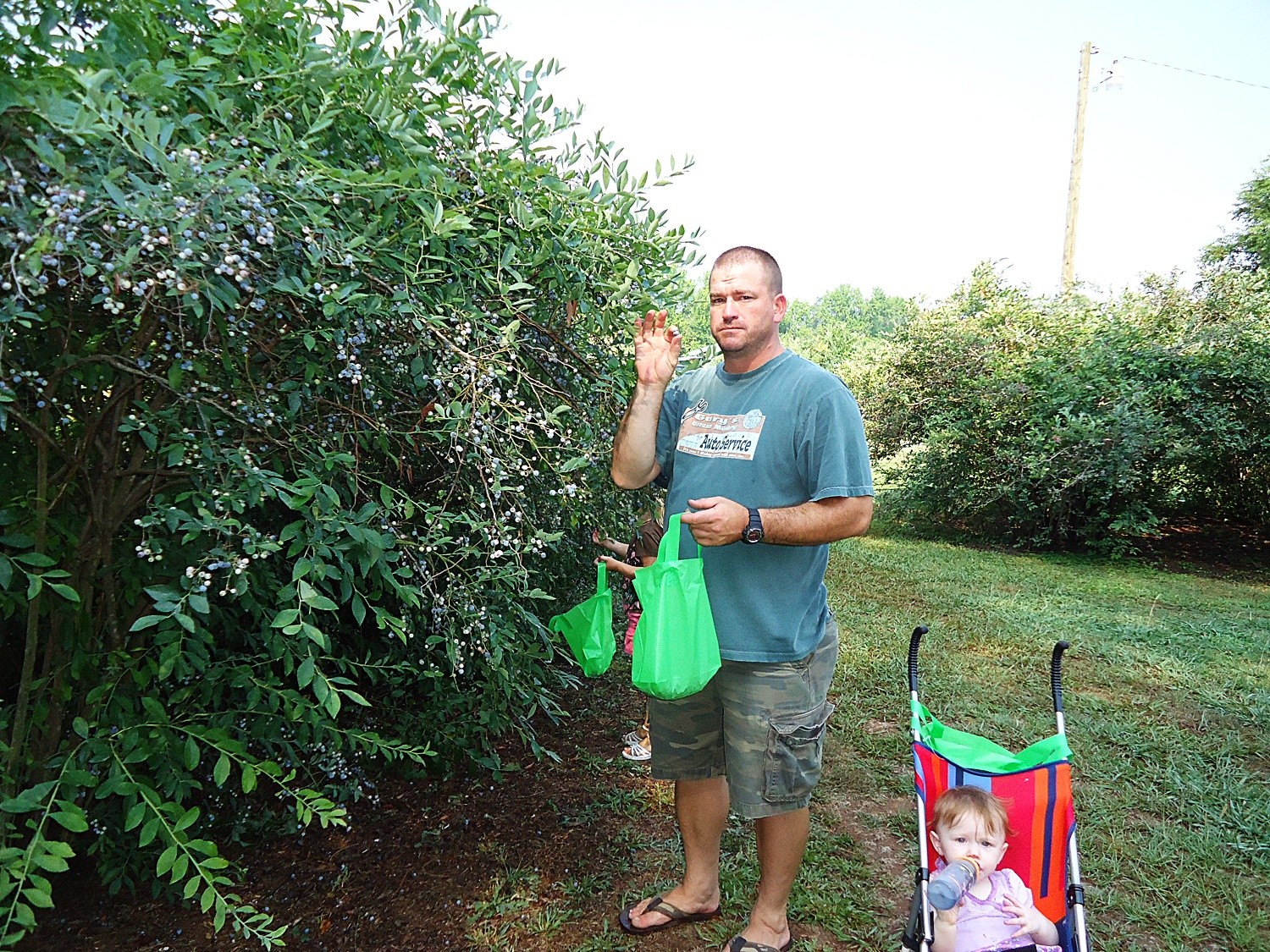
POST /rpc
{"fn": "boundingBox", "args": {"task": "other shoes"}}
[620,724,654,760]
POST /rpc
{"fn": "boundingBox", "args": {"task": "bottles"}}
[928,857,980,910]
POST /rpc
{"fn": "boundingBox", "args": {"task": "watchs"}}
[741,507,764,545]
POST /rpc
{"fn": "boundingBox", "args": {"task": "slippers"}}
[721,933,793,952]
[619,897,720,936]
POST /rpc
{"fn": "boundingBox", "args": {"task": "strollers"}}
[896,621,1089,952]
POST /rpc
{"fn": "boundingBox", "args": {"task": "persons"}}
[608,244,876,952]
[591,495,665,762]
[927,783,1063,952]
